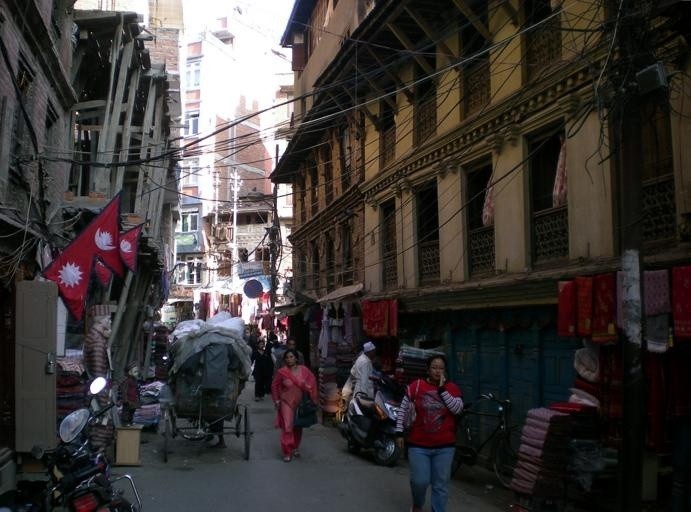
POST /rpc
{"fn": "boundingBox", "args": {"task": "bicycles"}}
[451,393,523,488]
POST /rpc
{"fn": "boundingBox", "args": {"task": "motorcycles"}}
[31,376,142,511]
[337,371,405,466]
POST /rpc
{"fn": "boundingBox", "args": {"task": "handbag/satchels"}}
[404,402,416,431]
[294,400,318,426]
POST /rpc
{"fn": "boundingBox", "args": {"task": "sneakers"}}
[293,448,300,457]
[283,454,290,461]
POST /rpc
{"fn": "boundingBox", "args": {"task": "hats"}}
[364,341,376,352]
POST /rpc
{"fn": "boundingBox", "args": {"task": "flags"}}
[93,227,145,289]
[44,190,125,320]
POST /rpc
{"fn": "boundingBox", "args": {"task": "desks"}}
[116,424,145,466]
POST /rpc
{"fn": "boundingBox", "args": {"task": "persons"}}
[395,355,464,512]
[242,324,305,400]
[271,349,317,463]
[351,341,377,400]
[84,303,113,448]
[104,325,112,339]
[122,359,140,426]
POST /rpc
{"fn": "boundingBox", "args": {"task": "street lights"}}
[248,144,279,332]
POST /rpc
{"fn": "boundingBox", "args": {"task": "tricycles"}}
[163,368,253,463]
